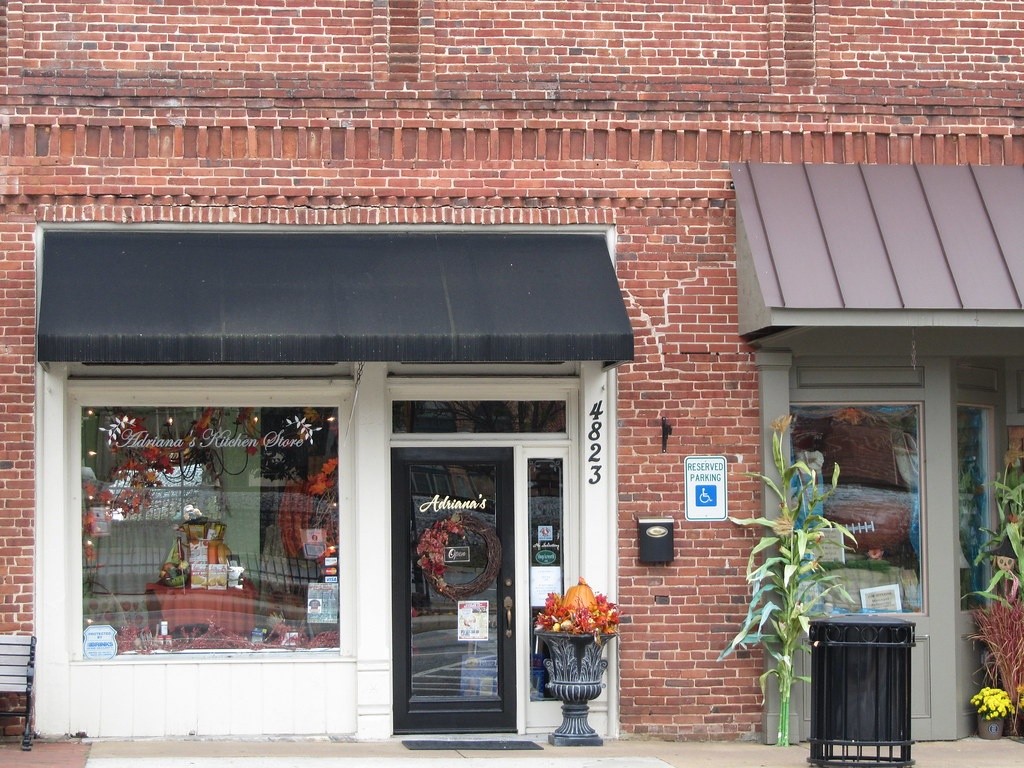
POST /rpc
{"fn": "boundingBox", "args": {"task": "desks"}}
[145,580,255,638]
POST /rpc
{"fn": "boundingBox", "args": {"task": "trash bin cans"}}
[809,618,915,768]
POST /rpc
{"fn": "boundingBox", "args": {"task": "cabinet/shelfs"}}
[806,612,917,767]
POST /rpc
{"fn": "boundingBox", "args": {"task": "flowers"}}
[970,686,1015,721]
[416,513,502,598]
[535,591,622,645]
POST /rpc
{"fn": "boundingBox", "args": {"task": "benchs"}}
[0,634,37,750]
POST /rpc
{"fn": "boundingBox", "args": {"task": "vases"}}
[534,630,617,747]
[977,714,1004,740]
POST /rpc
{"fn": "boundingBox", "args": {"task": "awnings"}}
[35,229,633,368]
